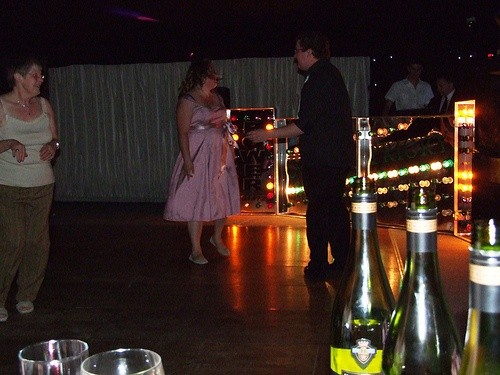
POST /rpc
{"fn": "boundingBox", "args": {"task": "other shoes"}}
[303,265,329,279]
[328,262,353,272]
[189,253,209,265]
[209,236,231,256]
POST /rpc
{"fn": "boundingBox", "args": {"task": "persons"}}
[382,56,434,116]
[163,63,241,264]
[247,30,355,278]
[422,70,458,115]
[0,56,60,322]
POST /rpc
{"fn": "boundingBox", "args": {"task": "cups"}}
[80,347,164,375]
[18,339,89,375]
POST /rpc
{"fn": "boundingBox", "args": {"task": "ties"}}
[440,96,448,117]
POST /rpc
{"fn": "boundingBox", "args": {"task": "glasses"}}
[17,69,45,79]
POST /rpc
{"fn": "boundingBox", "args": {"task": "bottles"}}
[329,182,397,375]
[458,215,500,375]
[381,189,463,375]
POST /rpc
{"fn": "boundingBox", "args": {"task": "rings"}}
[16,150,20,151]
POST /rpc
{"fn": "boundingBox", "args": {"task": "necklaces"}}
[14,87,32,120]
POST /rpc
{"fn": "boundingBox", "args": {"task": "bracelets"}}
[54,140,59,149]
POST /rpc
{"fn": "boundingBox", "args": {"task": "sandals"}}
[0,307,8,322]
[15,300,34,313]
